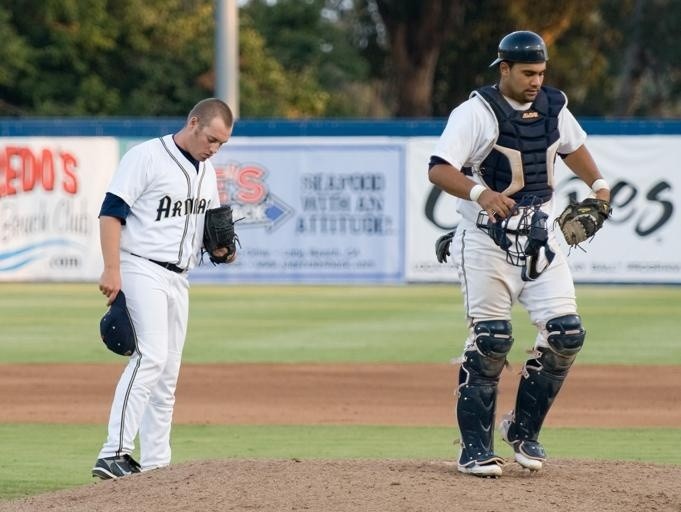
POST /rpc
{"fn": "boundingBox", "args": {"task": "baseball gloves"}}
[203,205,241,266]
[552,198,611,255]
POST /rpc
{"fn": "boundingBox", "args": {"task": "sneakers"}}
[457,460,501,477]
[92,454,141,479]
[499,421,543,470]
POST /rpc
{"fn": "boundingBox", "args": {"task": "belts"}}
[131,252,184,273]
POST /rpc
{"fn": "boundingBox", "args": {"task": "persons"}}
[90,98,235,480]
[427,30,613,479]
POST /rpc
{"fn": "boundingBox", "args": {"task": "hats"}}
[489,31,548,68]
[100,289,136,356]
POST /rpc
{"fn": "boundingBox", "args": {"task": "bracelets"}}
[469,183,486,202]
[591,178,610,194]
